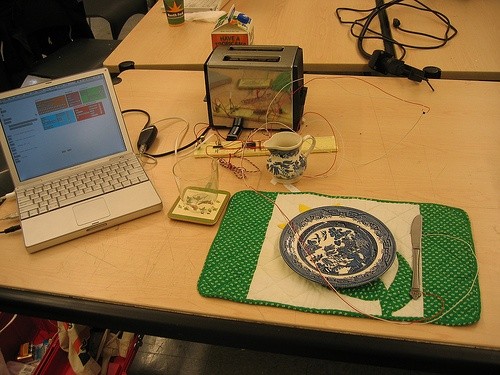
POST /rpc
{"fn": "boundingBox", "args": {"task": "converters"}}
[137,125,158,148]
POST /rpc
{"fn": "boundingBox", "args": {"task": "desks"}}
[104,0,500,82]
[0,62,500,374]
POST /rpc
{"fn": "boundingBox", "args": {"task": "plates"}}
[280,206,396,288]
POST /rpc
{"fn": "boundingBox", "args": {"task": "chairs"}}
[0,1,147,92]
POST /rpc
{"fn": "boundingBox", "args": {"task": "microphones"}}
[367,49,427,83]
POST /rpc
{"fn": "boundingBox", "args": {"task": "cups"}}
[163,0,184,26]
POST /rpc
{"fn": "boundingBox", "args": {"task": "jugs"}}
[262,133,316,184]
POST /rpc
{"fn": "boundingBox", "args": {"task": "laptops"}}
[0,68,163,256]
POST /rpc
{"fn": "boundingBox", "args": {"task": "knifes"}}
[410,215,422,299]
